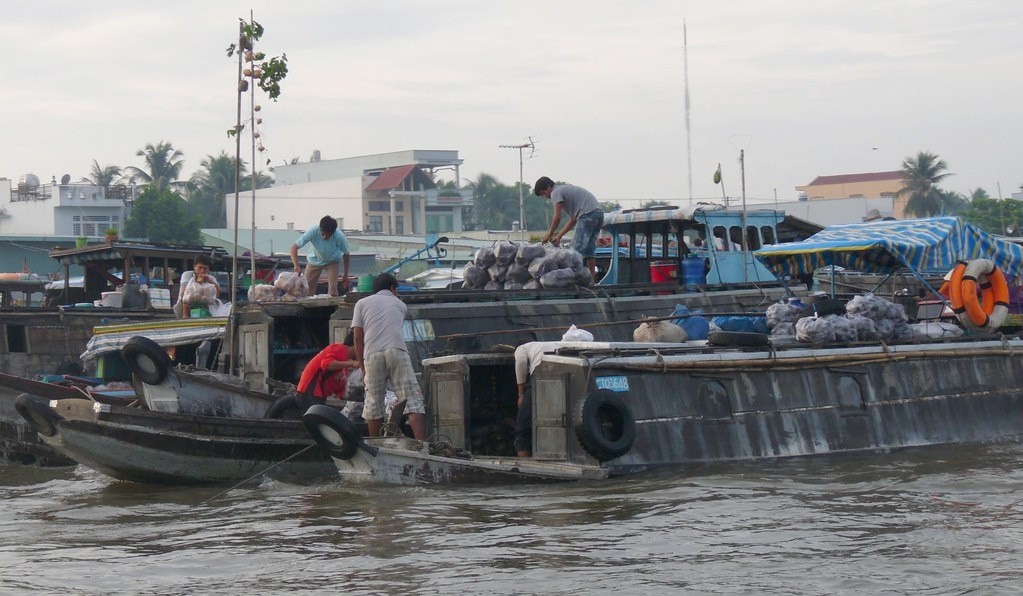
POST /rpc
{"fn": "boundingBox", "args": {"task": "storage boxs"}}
[145,288,171,309]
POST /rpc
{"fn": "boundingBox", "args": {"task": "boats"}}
[299,253,1023,473]
[2,205,1022,484]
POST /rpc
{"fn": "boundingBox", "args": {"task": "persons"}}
[350,273,426,440]
[289,215,350,296]
[535,177,604,280]
[297,328,365,410]
[174,256,222,320]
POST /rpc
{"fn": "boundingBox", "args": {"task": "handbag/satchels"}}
[462,240,593,289]
[247,283,278,303]
[182,273,217,304]
[766,293,912,343]
[273,272,309,297]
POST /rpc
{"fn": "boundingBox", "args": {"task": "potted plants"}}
[104,227,120,246]
[75,235,88,248]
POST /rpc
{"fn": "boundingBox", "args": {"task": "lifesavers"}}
[708,330,768,347]
[265,393,303,420]
[14,392,57,437]
[302,404,363,460]
[573,387,637,461]
[792,298,848,331]
[948,257,1010,331]
[122,335,169,386]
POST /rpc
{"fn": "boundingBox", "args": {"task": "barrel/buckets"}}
[357,275,376,292]
[650,264,677,294]
[102,292,122,308]
[681,253,705,291]
